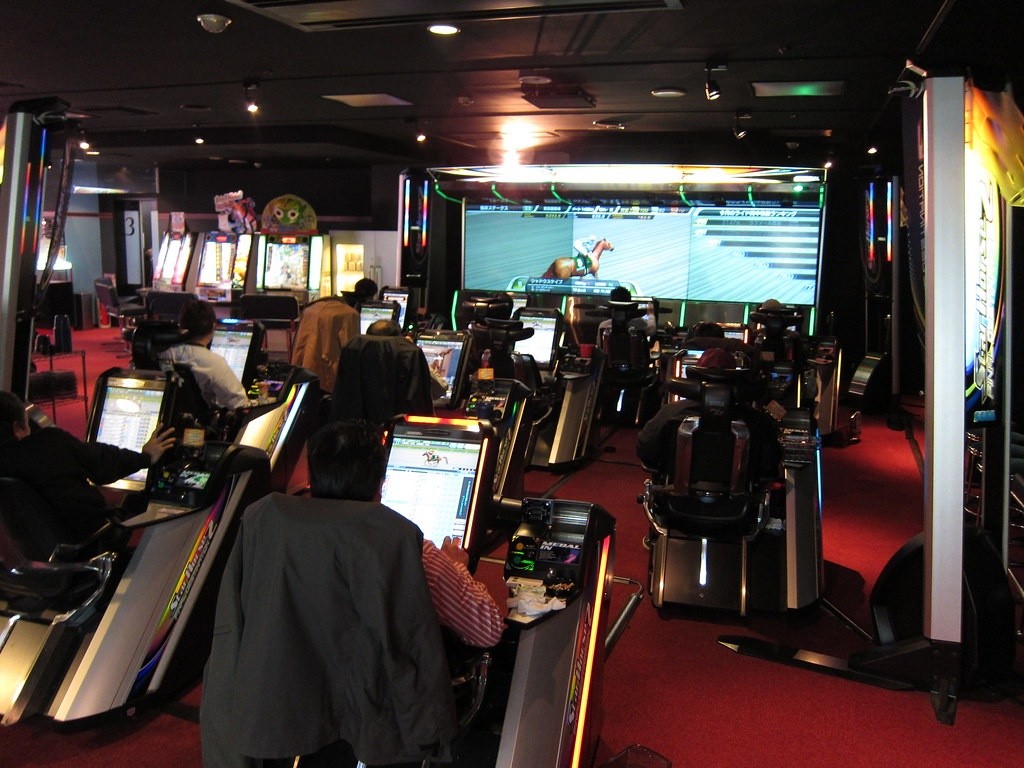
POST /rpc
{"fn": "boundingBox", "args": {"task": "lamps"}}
[521,88,597,109]
[197,15,232,33]
[703,60,728,100]
[404,117,426,142]
[905,52,929,78]
[734,113,753,140]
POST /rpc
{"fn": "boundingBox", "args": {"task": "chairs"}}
[202,493,493,768]
[0,337,237,729]
[94,279,145,334]
[635,307,826,626]
[287,295,673,469]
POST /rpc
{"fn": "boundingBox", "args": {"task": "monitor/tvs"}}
[85,286,800,564]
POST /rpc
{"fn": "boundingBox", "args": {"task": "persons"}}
[0,389,175,559]
[306,420,506,683]
[635,348,787,552]
[591,286,656,349]
[379,285,390,302]
[156,300,251,443]
[353,277,378,313]
[495,291,514,320]
[748,298,820,420]
[366,319,449,401]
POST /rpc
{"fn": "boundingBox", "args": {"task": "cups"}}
[54,314,71,353]
[34,334,52,354]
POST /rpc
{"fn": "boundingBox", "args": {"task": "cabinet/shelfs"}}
[29,350,89,425]
